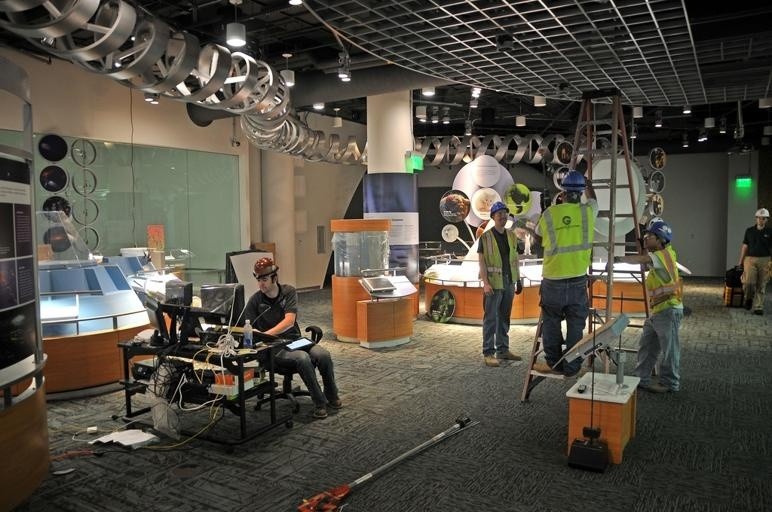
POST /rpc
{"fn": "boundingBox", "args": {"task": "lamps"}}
[759,98,772,108]
[681,134,690,150]
[684,105,691,115]
[516,100,526,127]
[338,51,352,83]
[654,110,662,128]
[333,108,342,129]
[225,22,246,48]
[432,105,439,123]
[416,89,427,122]
[534,95,546,107]
[423,87,436,97]
[279,55,294,87]
[633,104,643,118]
[442,106,450,125]
[470,97,478,108]
[735,174,753,188]
[704,117,715,128]
[465,109,472,137]
[311,100,324,110]
[720,127,726,133]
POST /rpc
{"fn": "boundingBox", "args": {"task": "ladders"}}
[522,88,657,403]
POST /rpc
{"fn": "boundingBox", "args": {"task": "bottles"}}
[243,320,252,349]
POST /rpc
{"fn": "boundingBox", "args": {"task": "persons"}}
[535,170,598,380]
[237,256,342,419]
[477,201,523,368]
[605,220,683,393]
[738,208,772,316]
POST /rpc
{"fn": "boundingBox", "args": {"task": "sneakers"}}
[533,362,555,374]
[496,352,521,361]
[484,354,499,367]
[646,382,679,393]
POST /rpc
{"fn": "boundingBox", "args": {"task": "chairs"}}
[254,327,322,414]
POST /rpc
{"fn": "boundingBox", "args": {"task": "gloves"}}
[605,256,619,271]
[515,280,522,295]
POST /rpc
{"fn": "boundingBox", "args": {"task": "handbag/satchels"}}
[725,268,743,288]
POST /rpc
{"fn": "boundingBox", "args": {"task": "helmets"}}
[253,257,279,279]
[560,170,587,191]
[646,221,672,242]
[755,208,769,217]
[491,202,509,218]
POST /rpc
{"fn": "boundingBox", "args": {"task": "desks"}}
[115,332,297,449]
[38,323,160,398]
[426,260,687,326]
[566,371,641,464]
[359,296,416,348]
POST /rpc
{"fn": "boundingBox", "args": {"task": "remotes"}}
[578,385,586,393]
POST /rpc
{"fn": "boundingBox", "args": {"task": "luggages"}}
[723,265,744,307]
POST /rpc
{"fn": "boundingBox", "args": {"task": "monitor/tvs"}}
[165,279,193,306]
[178,308,205,344]
[200,283,245,332]
[143,294,171,346]
[362,277,397,293]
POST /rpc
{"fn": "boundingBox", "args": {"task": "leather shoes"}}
[745,298,753,310]
[754,310,764,315]
[328,399,344,409]
[313,405,328,418]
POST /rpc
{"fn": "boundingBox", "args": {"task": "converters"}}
[87,426,97,433]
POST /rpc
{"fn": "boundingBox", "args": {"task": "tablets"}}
[285,337,315,352]
